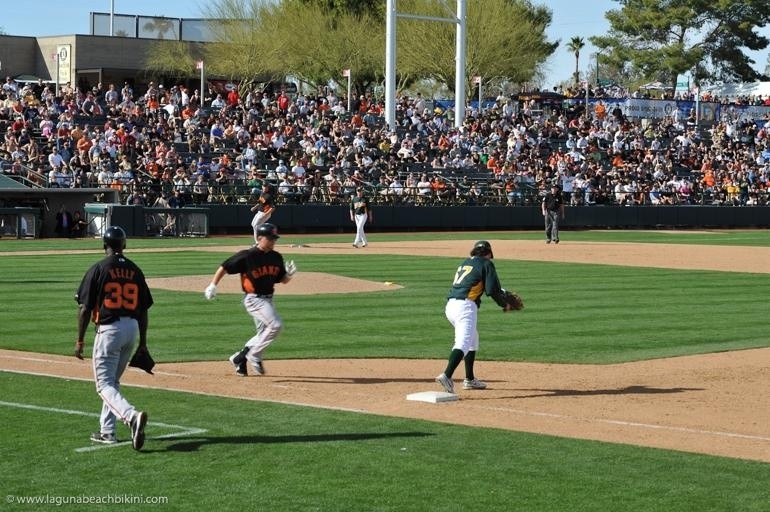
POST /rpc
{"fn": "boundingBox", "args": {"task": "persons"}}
[435,241,511,394]
[0,76,770,248]
[73,226,154,451]
[205,223,297,377]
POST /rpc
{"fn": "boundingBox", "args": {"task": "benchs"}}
[1,91,728,204]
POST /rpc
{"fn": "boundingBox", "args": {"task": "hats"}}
[475,240,494,259]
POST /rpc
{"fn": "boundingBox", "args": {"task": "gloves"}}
[285,260,297,275]
[205,283,217,300]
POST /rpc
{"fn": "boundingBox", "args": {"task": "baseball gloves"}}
[502,290,524,313]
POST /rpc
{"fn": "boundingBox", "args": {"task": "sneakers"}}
[435,372,455,394]
[246,353,264,375]
[90,431,116,444]
[463,377,486,390]
[230,352,248,376]
[129,412,147,450]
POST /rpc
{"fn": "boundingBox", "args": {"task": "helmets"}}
[104,225,127,250]
[256,222,280,240]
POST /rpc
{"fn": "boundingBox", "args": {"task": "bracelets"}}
[77,342,83,345]
